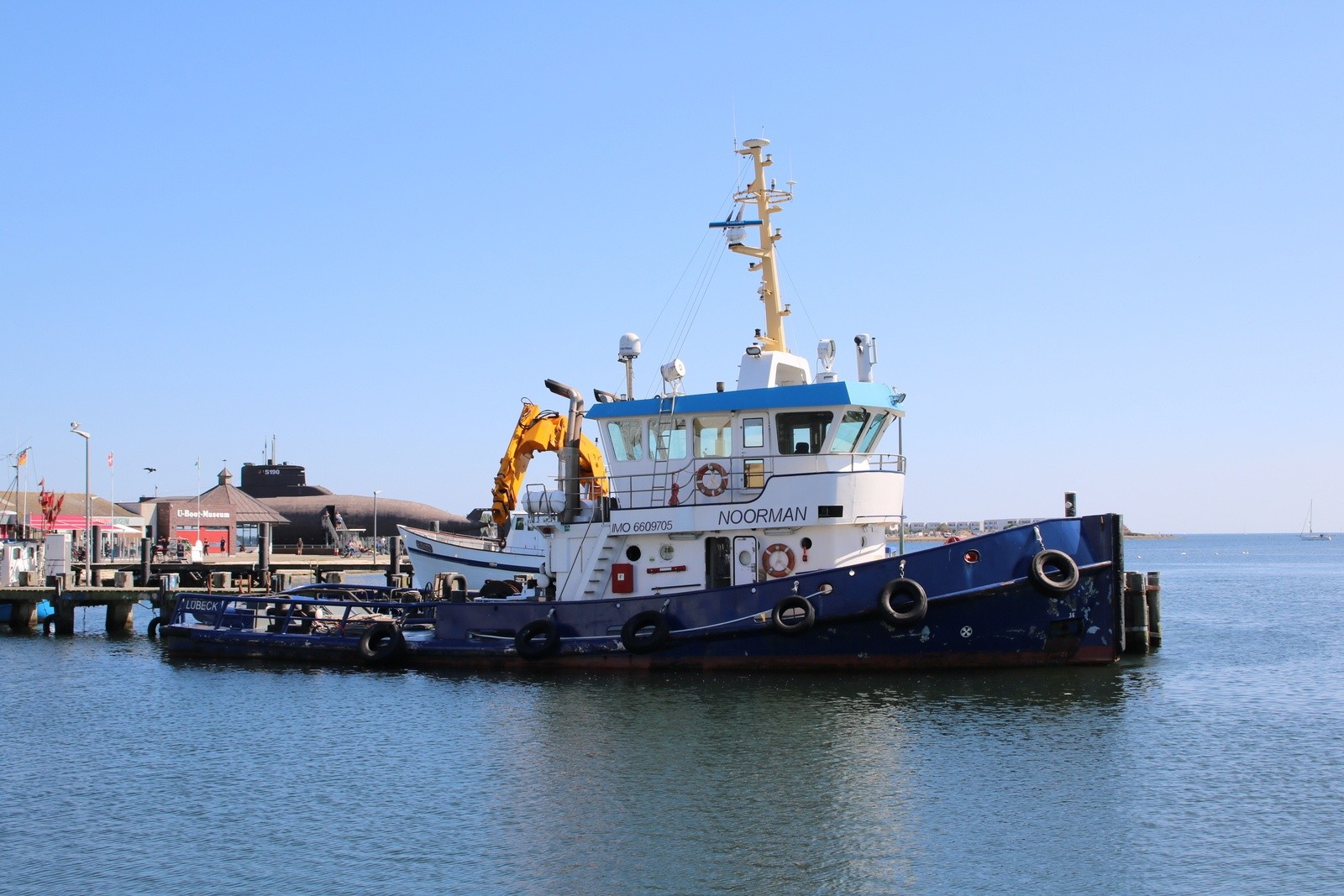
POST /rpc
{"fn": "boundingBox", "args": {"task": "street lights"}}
[69,429,91,586]
[374,490,382,565]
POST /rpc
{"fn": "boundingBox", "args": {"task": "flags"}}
[36,478,65,534]
[108,454,113,467]
[195,458,200,468]
[18,448,27,464]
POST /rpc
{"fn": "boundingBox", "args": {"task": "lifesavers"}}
[1030,549,1080,594]
[878,577,928,627]
[696,462,728,497]
[147,617,160,637]
[619,610,670,654]
[762,543,795,578]
[43,613,56,635]
[514,619,560,660]
[772,595,815,636]
[358,622,405,664]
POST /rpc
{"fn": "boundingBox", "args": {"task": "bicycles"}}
[338,545,362,558]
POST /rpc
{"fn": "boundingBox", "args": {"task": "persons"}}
[104,541,137,557]
[220,536,227,556]
[203,538,211,557]
[150,536,171,562]
[325,511,389,557]
[297,538,304,556]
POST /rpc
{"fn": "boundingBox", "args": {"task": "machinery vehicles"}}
[489,395,610,551]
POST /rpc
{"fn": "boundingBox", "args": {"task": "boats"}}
[394,482,564,599]
[130,121,1165,677]
